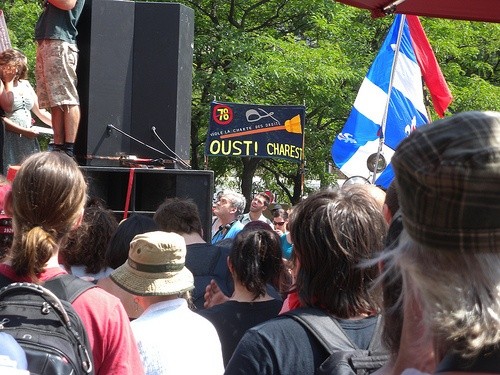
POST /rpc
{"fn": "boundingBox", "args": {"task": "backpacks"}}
[283,305,390,375]
[0,273,102,375]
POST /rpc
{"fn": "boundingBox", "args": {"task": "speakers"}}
[74,0,195,168]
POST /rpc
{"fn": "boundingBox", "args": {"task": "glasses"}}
[273,221,285,226]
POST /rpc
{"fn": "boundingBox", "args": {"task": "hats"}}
[109,231,195,296]
[270,205,282,215]
[391,109,500,250]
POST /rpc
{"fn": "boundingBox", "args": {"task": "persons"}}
[0,47,53,174]
[223,188,392,375]
[33,0,85,161]
[0,151,146,375]
[0,110,500,375]
[109,231,225,375]
[195,225,284,369]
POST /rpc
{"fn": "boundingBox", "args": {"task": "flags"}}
[332,13,452,190]
